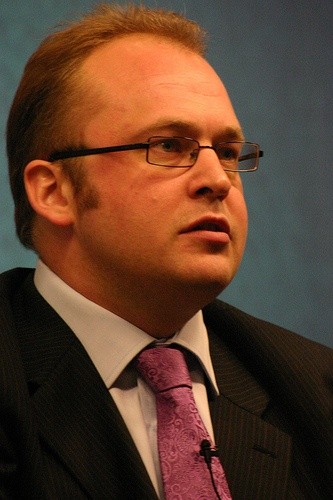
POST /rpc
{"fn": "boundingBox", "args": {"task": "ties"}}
[133,347,233,500]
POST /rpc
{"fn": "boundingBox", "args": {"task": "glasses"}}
[48,135,264,172]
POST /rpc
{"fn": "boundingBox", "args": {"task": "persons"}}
[0,0,333,500]
